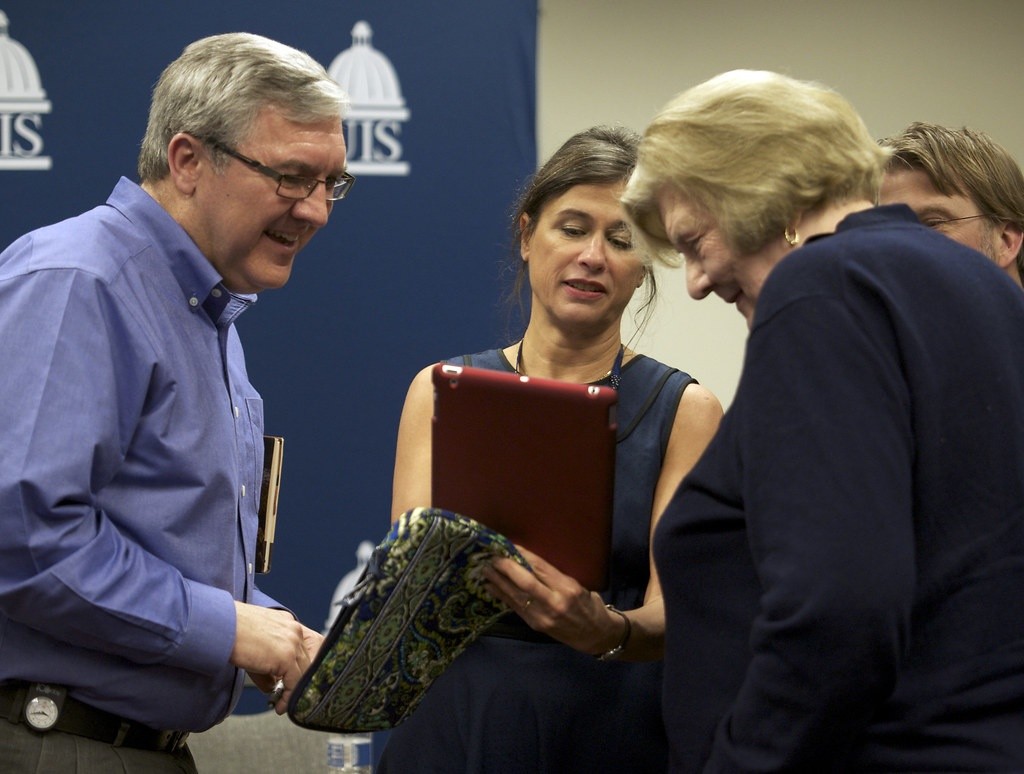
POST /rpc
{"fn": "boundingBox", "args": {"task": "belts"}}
[0,680,190,754]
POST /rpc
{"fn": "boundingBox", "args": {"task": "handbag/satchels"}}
[287,505,539,735]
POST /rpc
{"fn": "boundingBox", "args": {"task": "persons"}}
[875,119,1024,295]
[375,127,723,774]
[620,69,1024,774]
[0,28,355,774]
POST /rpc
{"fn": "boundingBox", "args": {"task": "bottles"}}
[327,732,372,774]
[325,540,376,636]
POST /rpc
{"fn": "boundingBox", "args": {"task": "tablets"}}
[430,365,617,596]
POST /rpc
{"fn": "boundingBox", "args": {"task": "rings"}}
[524,599,531,611]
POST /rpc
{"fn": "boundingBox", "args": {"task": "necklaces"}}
[512,339,623,390]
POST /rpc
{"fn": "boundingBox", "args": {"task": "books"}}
[254,437,284,576]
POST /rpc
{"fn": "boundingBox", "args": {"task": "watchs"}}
[592,603,631,663]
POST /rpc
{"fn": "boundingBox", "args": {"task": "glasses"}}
[202,136,357,201]
[916,210,993,231]
